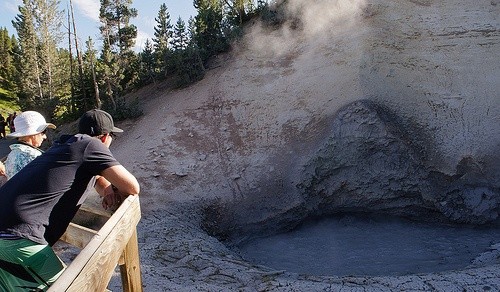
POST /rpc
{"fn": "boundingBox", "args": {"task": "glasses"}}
[100,132,113,139]
[42,129,47,134]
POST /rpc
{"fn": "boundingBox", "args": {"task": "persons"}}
[7,113,14,133]
[5,110,56,181]
[0,109,140,292]
[0,113,5,140]
[13,112,17,119]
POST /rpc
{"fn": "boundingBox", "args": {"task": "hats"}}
[79,109,124,132]
[6,110,56,137]
